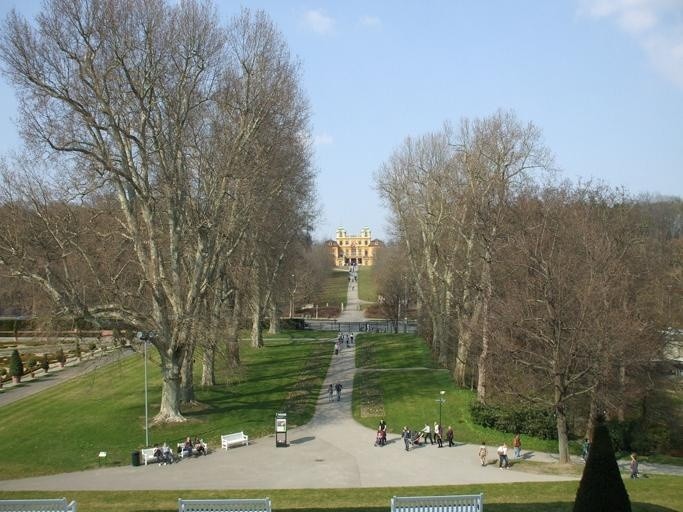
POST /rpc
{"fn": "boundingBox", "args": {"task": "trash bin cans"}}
[132,451,139,466]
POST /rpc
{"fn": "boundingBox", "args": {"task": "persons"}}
[152,444,164,466]
[400,421,454,451]
[333,331,353,357]
[335,382,342,401]
[327,384,334,402]
[629,452,639,478]
[512,433,521,456]
[376,432,381,442]
[377,419,386,446]
[184,436,194,457]
[478,441,486,466]
[194,436,206,456]
[497,441,508,469]
[161,442,174,464]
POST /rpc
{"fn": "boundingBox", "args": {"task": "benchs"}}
[176,439,208,459]
[141,445,174,466]
[221,431,249,450]
[390,493,484,512]
[177,496,272,512]
[0,496,76,512]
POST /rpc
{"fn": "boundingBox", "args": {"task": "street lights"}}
[438,390,446,427]
[135,329,155,448]
